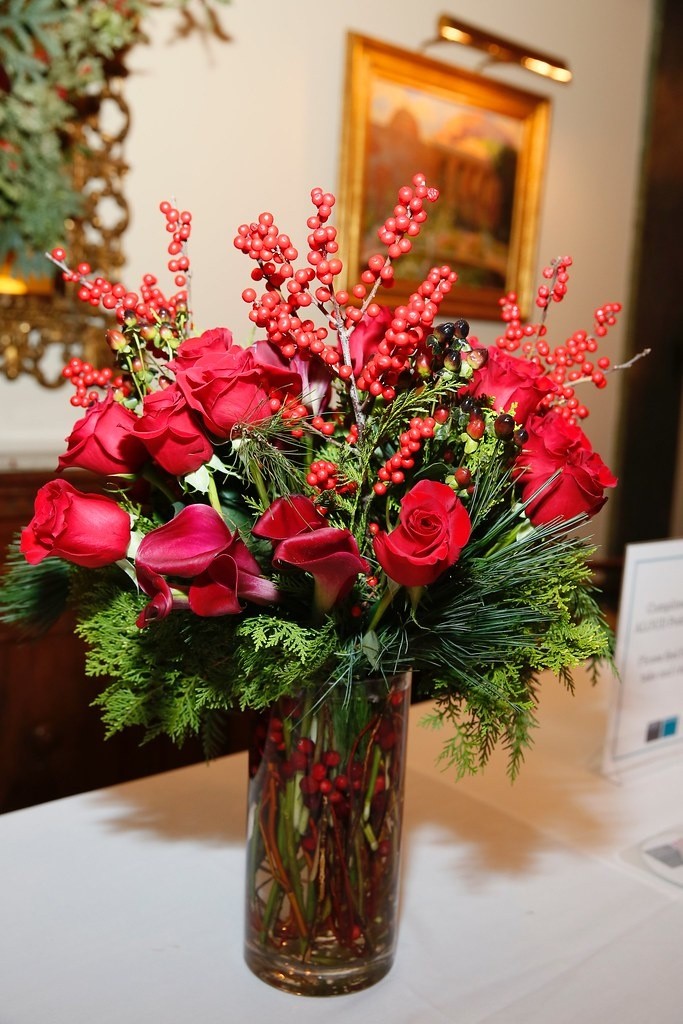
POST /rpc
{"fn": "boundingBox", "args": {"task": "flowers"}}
[0,173,654,966]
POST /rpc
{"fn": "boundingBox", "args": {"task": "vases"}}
[237,666,412,1000]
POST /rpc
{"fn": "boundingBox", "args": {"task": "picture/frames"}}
[330,24,553,326]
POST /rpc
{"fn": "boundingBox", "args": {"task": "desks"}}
[0,652,683,1022]
